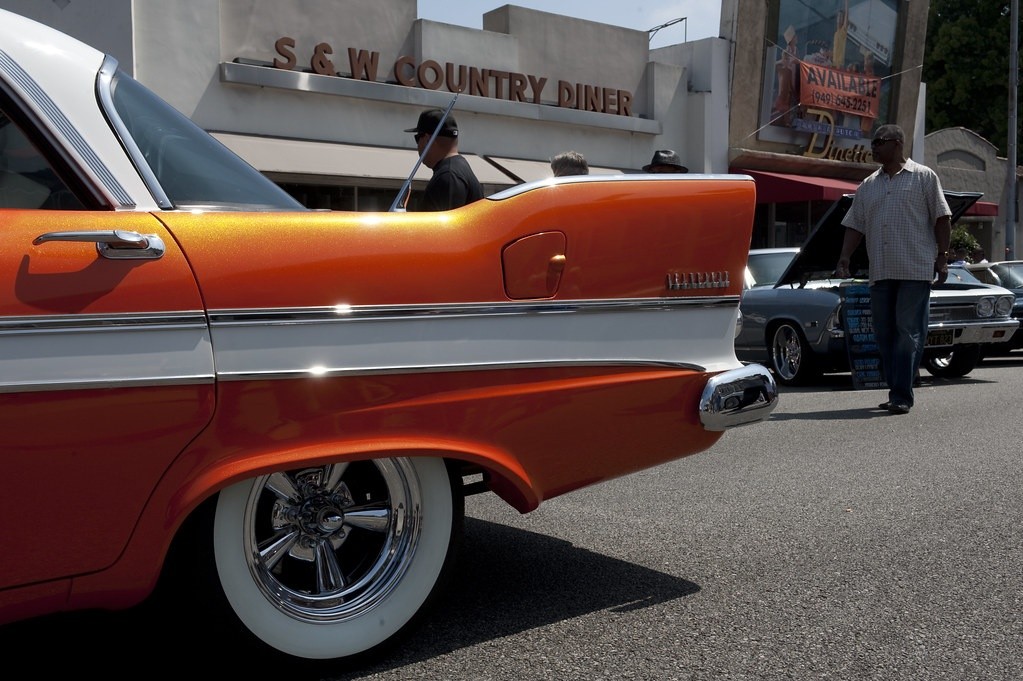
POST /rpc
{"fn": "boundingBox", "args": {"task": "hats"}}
[403,108,458,138]
[641,149,689,173]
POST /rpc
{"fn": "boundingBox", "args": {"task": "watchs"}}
[938,252,949,257]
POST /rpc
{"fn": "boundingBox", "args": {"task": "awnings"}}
[488,155,642,185]
[212,133,517,197]
[736,170,998,216]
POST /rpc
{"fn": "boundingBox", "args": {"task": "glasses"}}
[871,138,901,147]
[413,133,434,144]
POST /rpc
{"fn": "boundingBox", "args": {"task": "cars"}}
[0,8,778,681]
[944,259,1023,355]
[735,186,1021,389]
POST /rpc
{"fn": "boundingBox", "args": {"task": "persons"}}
[835,124,954,415]
[551,152,589,177]
[403,107,482,212]
[642,150,688,173]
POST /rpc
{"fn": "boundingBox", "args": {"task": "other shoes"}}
[878,402,893,410]
[889,404,910,415]
